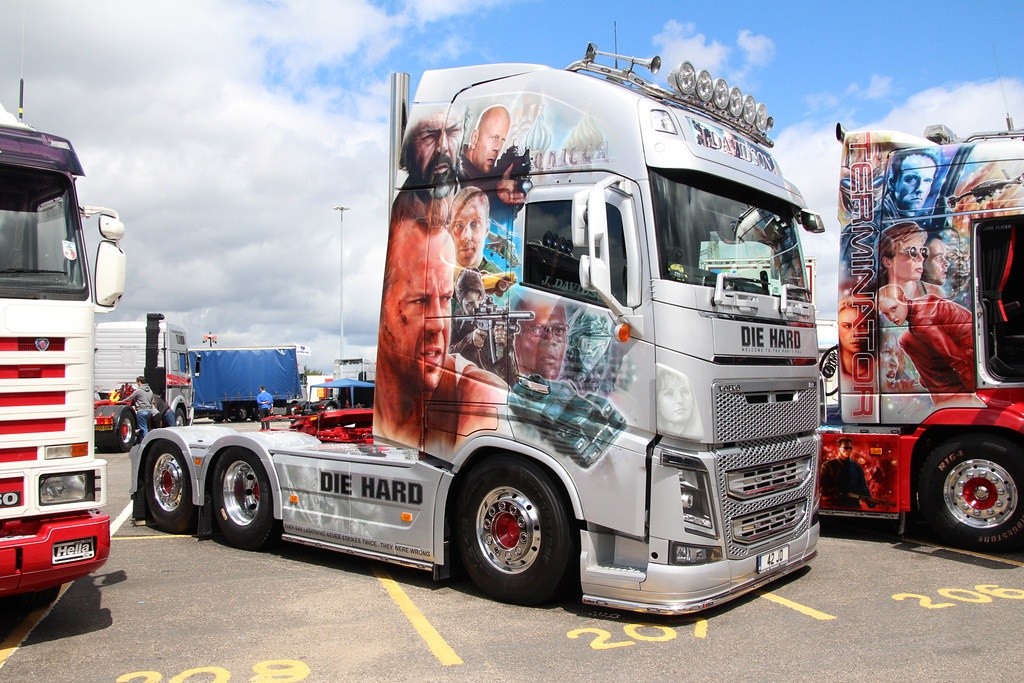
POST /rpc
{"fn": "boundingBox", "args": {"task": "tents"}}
[308,377,375,414]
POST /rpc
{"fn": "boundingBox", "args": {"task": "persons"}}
[116,376,177,437]
[649,368,703,438]
[257,386,273,431]
[374,91,567,465]
[818,436,875,510]
[838,148,976,403]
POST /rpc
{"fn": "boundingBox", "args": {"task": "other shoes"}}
[259,428,271,432]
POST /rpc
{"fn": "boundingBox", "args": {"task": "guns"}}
[453,296,535,363]
[493,138,533,220]
[818,490,897,508]
[924,144,975,233]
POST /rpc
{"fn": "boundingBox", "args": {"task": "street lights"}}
[333,206,351,358]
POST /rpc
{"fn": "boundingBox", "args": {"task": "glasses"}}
[525,321,570,344]
[841,445,854,451]
[896,245,930,260]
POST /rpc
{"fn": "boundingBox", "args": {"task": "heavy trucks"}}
[0,79,378,611]
[816,46,1024,554]
[131,17,821,615]
